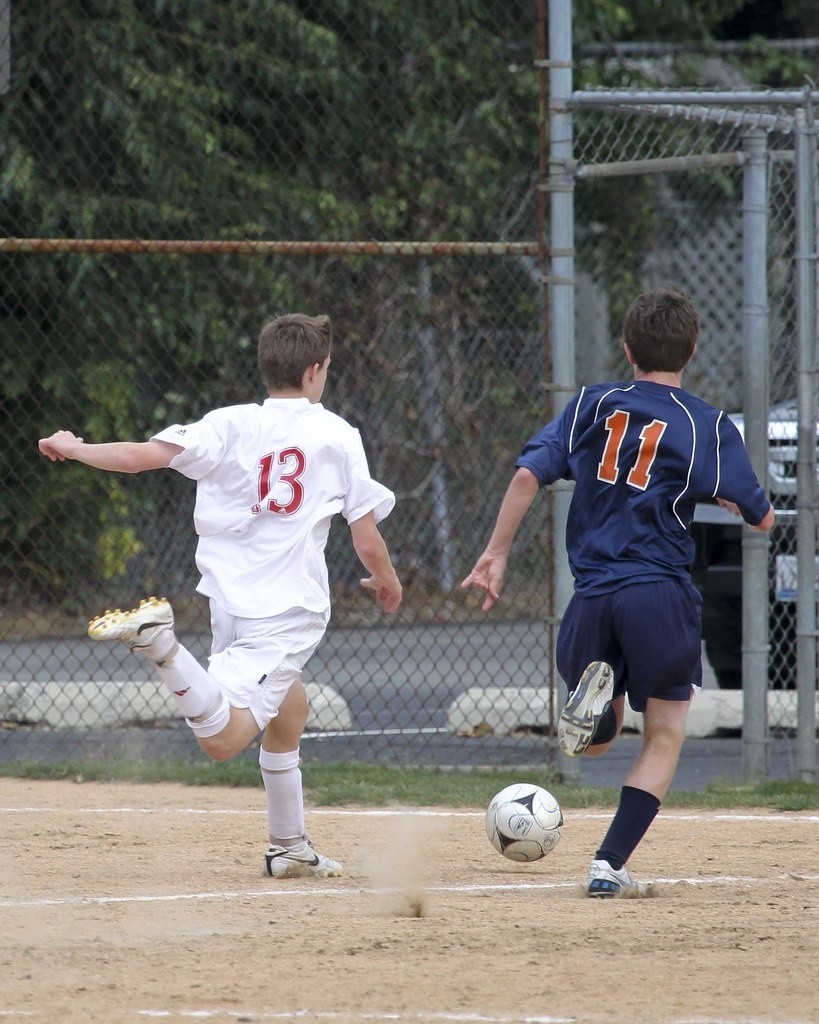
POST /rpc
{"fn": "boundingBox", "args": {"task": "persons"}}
[458,290,775,896]
[39,313,403,880]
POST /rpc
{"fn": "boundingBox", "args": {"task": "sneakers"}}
[586,859,639,895]
[265,841,343,879]
[557,659,614,757]
[87,596,175,655]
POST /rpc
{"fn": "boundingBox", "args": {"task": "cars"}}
[687,395,817,695]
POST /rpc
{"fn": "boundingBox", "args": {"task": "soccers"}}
[481,779,569,866]
